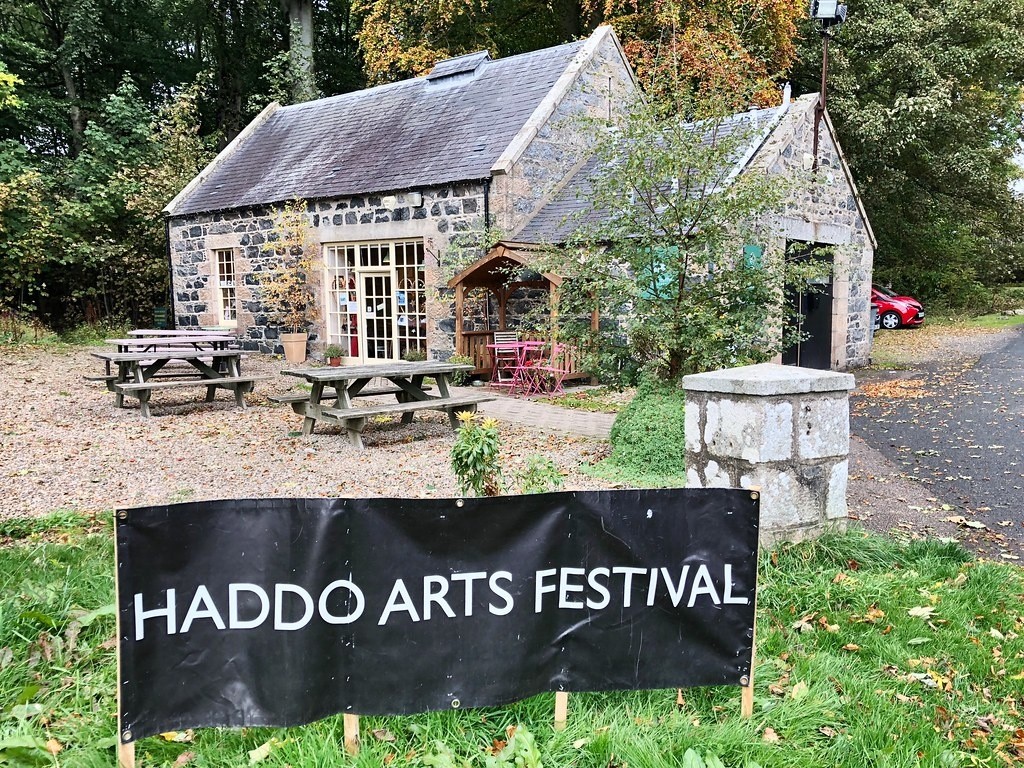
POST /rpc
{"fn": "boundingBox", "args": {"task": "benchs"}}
[90,343,496,419]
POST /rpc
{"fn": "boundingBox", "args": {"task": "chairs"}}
[485,331,578,399]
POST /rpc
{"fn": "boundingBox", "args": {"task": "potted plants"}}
[252,195,344,366]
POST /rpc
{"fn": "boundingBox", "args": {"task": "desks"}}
[485,345,524,396]
[127,329,234,353]
[91,349,261,417]
[102,336,236,378]
[280,359,476,449]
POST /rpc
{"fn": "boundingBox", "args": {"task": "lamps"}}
[407,191,424,209]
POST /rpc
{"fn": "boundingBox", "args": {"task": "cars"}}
[871,282,924,329]
